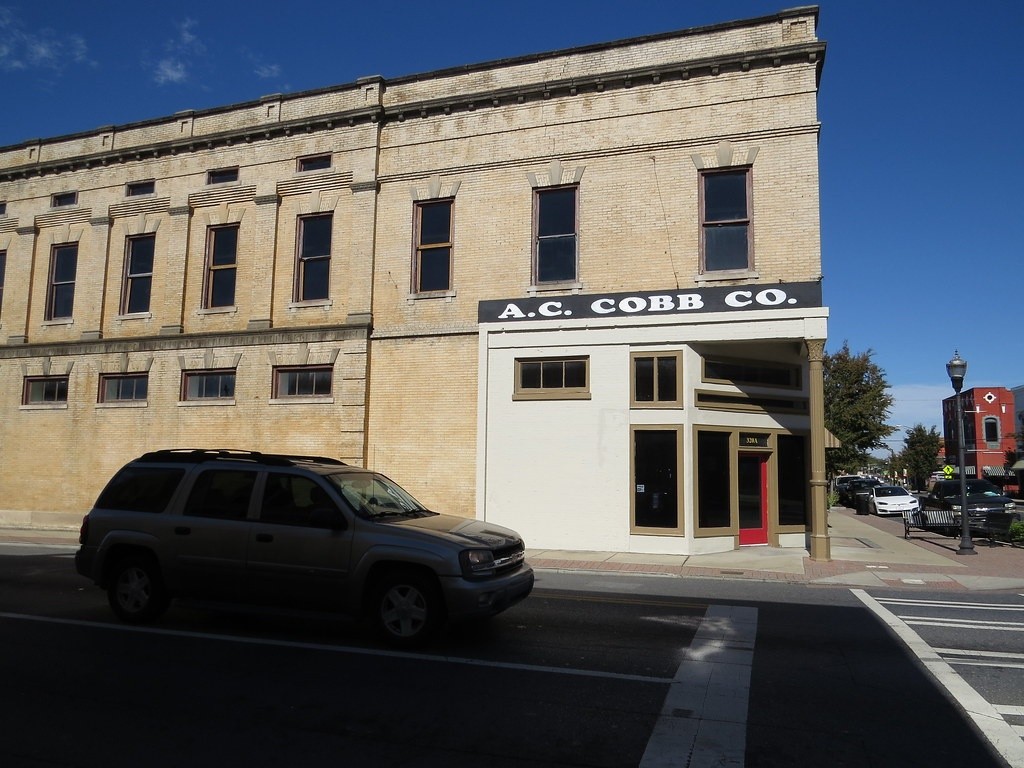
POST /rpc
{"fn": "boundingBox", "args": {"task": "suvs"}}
[75,448,535,653]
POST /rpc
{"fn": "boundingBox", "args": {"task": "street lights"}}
[946,349,979,555]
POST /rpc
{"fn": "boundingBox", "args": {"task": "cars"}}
[867,485,921,516]
[835,473,884,508]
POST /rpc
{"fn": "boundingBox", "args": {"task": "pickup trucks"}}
[918,478,1015,537]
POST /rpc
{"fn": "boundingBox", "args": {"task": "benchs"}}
[901,509,960,540]
